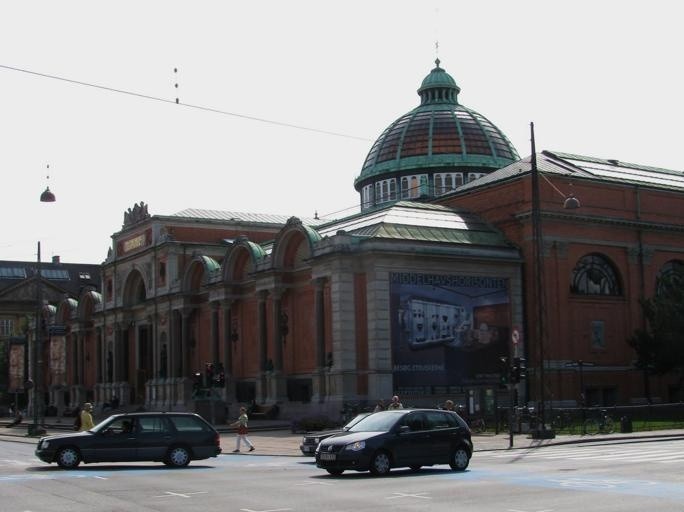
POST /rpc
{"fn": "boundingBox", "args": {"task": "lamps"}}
[39,164,56,202]
[564,172,581,209]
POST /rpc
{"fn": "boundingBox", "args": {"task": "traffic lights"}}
[217,369,225,388]
[496,355,510,383]
[194,372,201,389]
[513,355,527,382]
[206,362,214,371]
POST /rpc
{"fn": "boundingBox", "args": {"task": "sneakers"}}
[250,447,255,451]
[233,449,240,452]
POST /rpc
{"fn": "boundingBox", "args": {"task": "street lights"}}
[530,122,579,439]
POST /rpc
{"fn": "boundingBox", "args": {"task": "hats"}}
[84,403,91,407]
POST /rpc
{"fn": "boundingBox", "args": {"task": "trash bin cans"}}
[621,416,632,433]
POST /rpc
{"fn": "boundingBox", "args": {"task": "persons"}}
[7,400,28,417]
[387,395,403,411]
[77,402,95,433]
[109,420,130,435]
[441,399,454,411]
[374,398,386,413]
[228,406,255,453]
[62,395,119,418]
[135,401,146,412]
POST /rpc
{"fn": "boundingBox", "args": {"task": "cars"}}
[300,411,376,456]
[313,408,472,475]
[35,411,221,467]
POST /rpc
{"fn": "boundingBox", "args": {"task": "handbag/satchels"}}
[239,425,248,434]
[73,415,81,431]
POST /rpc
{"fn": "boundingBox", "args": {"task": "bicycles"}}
[583,404,615,434]
